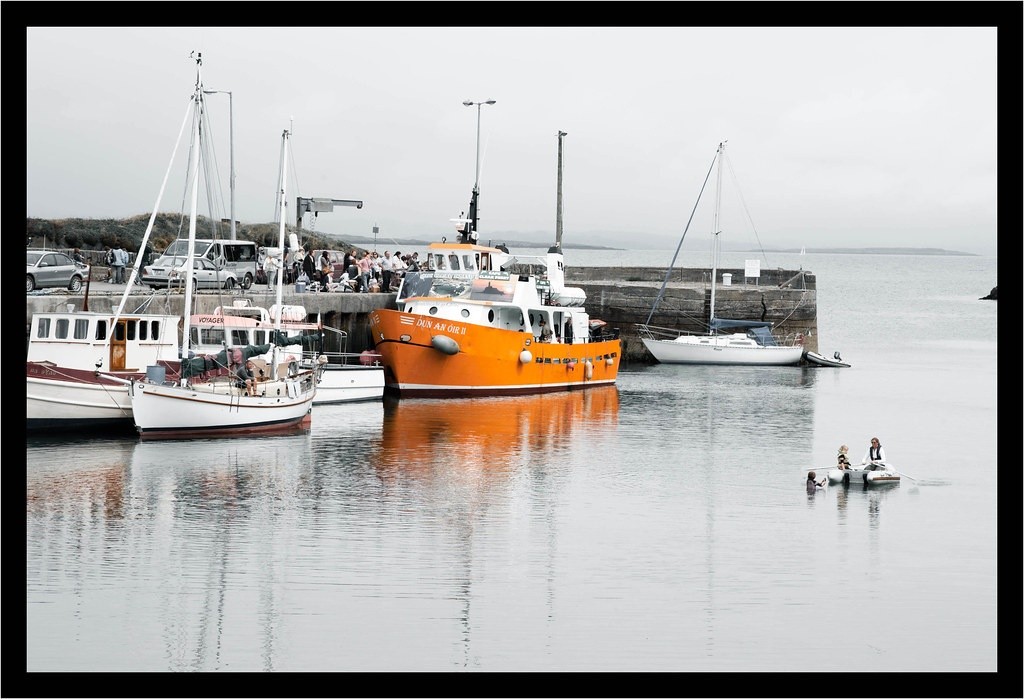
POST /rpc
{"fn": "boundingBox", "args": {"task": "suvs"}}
[304,247,346,279]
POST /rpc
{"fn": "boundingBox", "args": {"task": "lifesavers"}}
[285,355,296,362]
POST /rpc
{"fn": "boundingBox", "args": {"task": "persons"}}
[564,318,573,344]
[73,248,85,262]
[863,437,885,470]
[258,244,289,291]
[341,250,418,293]
[293,245,335,292]
[837,445,852,469]
[476,257,479,270]
[537,320,553,343]
[235,360,258,397]
[137,239,152,285]
[806,472,826,491]
[104,242,130,284]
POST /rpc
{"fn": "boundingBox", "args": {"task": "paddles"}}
[873,462,916,482]
[804,463,861,473]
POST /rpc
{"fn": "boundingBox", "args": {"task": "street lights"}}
[202,87,238,242]
[462,98,499,245]
[554,130,567,248]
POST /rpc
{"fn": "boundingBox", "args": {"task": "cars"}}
[140,256,238,290]
[26,250,90,293]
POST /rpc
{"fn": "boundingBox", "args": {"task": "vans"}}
[153,236,257,290]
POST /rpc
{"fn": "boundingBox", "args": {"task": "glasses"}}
[872,441,877,443]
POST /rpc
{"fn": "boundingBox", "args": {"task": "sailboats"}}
[628,141,805,366]
[94,48,349,440]
[188,118,386,405]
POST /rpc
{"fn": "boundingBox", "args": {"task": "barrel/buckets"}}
[181,379,187,388]
[147,366,165,386]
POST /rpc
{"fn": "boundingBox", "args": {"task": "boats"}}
[801,348,851,369]
[826,458,901,486]
[23,255,185,435]
[367,187,626,399]
